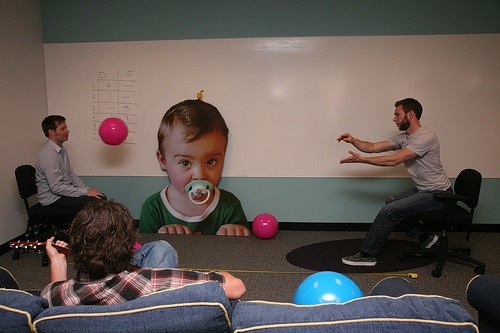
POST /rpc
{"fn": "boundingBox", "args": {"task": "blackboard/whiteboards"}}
[40,33,500,180]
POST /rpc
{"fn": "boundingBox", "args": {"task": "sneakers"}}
[342,252,377,267]
[415,233,439,257]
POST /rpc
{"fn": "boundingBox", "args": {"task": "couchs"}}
[0,267,480,333]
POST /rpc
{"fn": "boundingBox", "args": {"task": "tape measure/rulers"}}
[175,268,418,278]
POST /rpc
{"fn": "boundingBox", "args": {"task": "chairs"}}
[12,165,71,267]
[391,169,486,277]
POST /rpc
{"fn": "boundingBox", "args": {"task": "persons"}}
[36,115,108,225]
[40,199,246,307]
[137,99,253,236]
[337,98,455,266]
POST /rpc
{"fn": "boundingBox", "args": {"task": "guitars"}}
[9,240,141,273]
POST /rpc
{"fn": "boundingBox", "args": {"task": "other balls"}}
[252,213,278,239]
[98,117,130,146]
[293,271,365,305]
